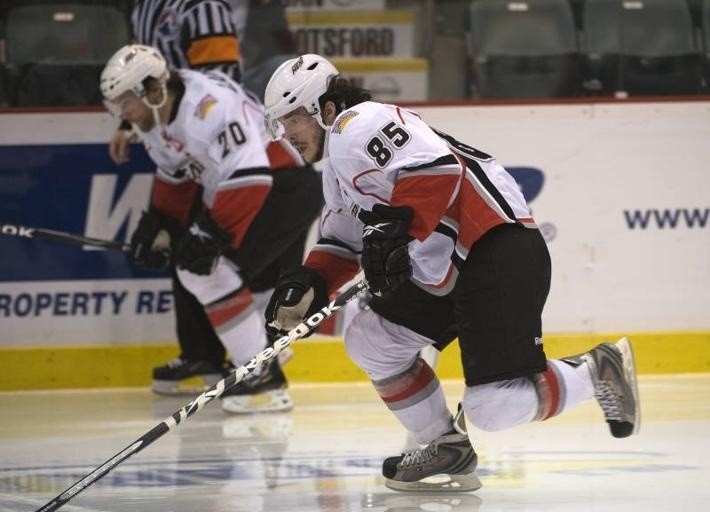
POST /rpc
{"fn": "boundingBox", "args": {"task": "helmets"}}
[262,53,341,143]
[99,44,171,123]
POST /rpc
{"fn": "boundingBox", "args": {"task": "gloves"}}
[172,206,233,276]
[129,204,182,273]
[264,263,329,340]
[357,203,414,301]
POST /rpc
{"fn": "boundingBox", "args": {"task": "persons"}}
[264,53,635,482]
[101,44,326,397]
[109,0,242,380]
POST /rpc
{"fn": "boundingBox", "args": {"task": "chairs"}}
[4,0,703,103]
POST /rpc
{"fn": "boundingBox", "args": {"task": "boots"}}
[383,402,477,483]
[558,340,636,437]
[152,353,225,382]
[220,337,289,397]
